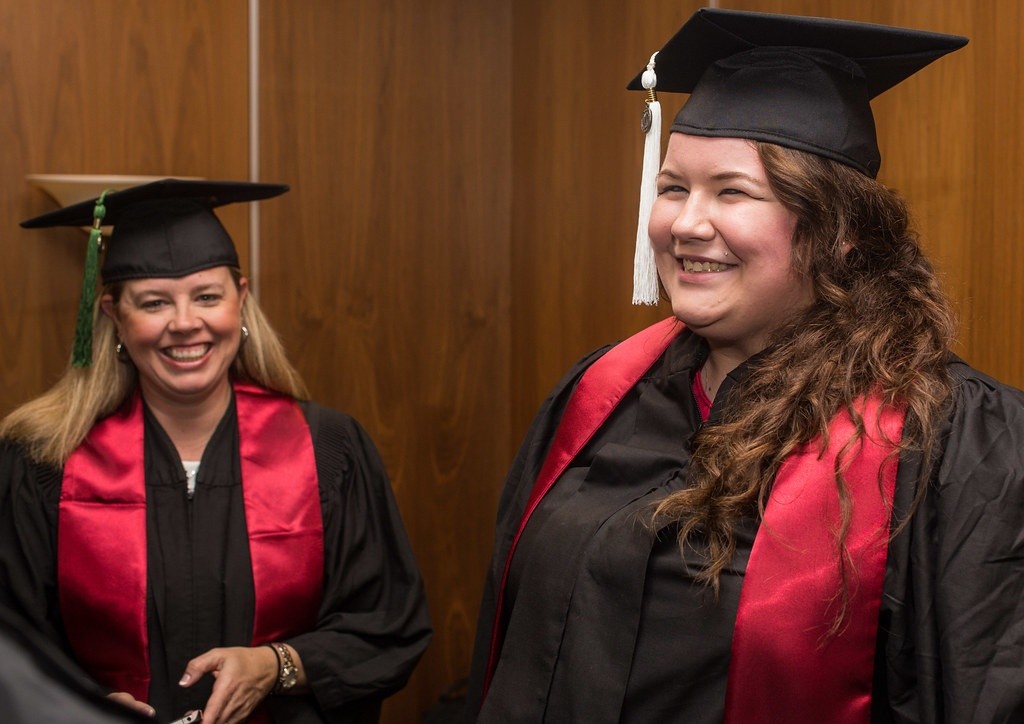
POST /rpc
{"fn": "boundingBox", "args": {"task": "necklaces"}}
[704,362,708,393]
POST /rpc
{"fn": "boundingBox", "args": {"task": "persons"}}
[451,9,1023,724]
[0,179,432,724]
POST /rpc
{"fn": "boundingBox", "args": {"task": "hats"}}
[627,7,969,308]
[21,178,289,369]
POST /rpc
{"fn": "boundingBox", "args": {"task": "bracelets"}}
[265,644,281,697]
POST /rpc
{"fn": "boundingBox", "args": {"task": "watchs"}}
[274,641,298,696]
